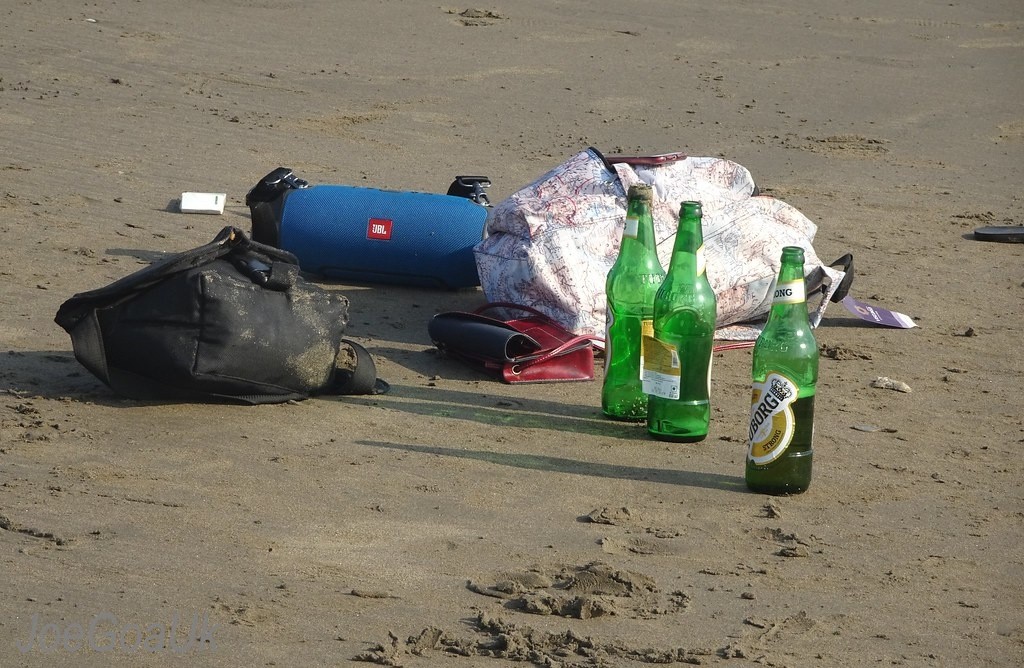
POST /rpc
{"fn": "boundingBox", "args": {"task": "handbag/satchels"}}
[244,166,495,288]
[428,302,607,384]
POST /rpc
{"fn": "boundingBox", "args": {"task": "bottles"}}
[601,184,666,421]
[745,246,818,496]
[644,201,717,444]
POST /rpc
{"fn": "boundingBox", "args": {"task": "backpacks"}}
[51,227,390,405]
[472,148,855,342]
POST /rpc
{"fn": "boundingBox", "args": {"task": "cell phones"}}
[603,152,686,165]
